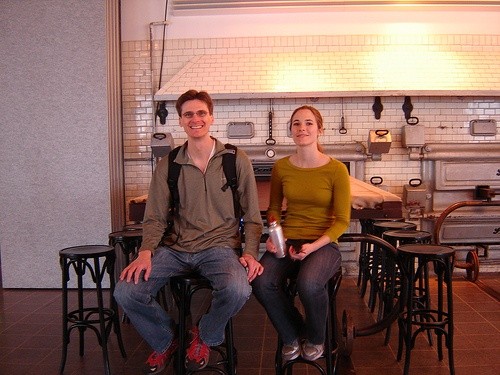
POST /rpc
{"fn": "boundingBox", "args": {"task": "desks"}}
[257,175,402,359]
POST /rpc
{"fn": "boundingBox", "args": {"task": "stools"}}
[59,224,144,375]
[170,274,236,375]
[357,217,457,375]
[275,266,342,375]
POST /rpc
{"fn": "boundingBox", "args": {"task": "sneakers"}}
[300,338,325,362]
[143,334,181,374]
[185,325,209,371]
[282,340,301,361]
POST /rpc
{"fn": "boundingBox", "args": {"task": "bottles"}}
[266,211,288,259]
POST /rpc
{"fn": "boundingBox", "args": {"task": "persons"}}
[251,105,352,360]
[114,89,264,375]
